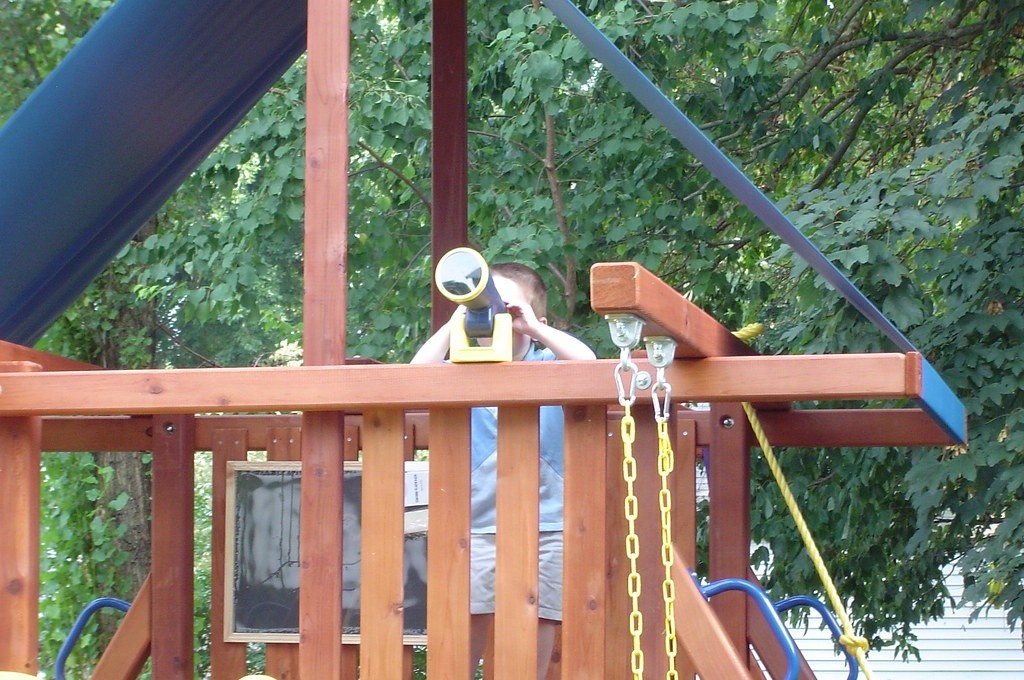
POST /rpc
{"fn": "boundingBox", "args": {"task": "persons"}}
[409,262,597,680]
